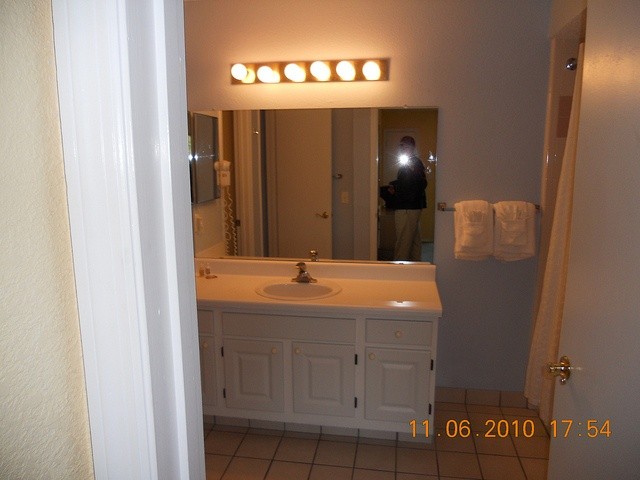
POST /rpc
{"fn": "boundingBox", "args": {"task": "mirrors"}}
[193,112,222,206]
[189,107,439,266]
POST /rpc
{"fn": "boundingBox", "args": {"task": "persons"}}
[380,135,428,262]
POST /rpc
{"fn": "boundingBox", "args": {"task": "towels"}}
[492,200,536,262]
[451,199,494,262]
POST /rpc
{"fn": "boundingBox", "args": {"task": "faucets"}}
[309,247,319,261]
[293,261,314,281]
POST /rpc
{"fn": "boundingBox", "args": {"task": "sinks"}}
[259,281,335,300]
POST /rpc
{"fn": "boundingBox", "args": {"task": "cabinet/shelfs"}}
[364,319,434,431]
[196,309,219,408]
[220,310,360,419]
